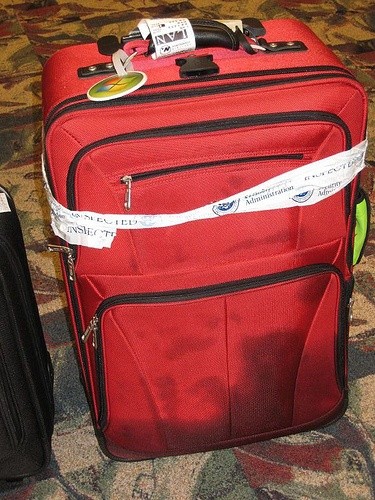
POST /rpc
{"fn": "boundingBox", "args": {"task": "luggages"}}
[42,19,368,462]
[0,182,55,479]
